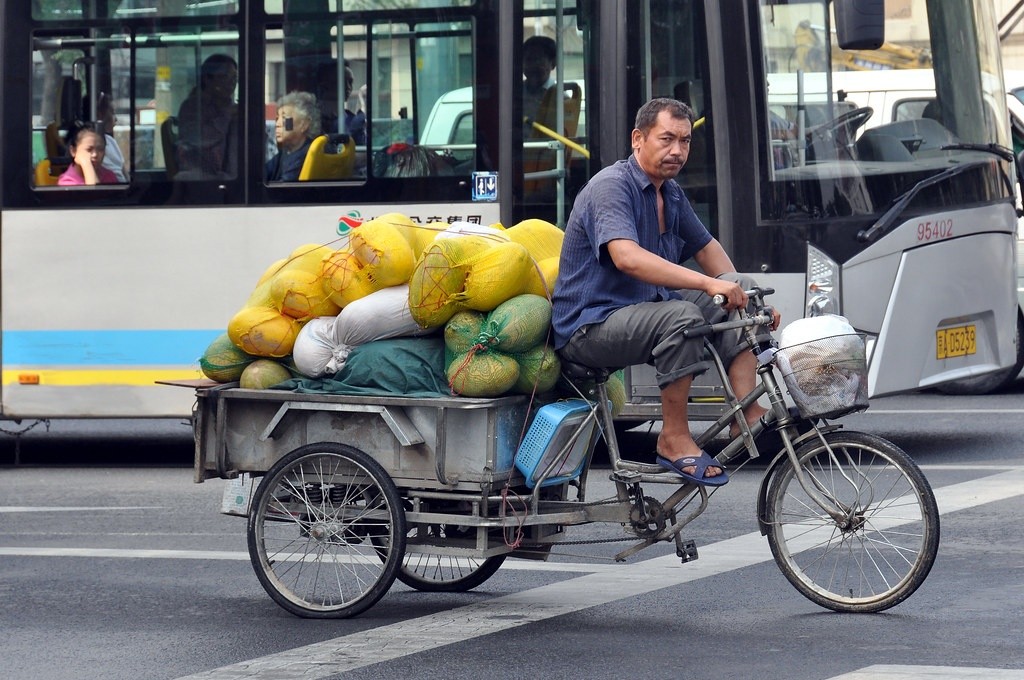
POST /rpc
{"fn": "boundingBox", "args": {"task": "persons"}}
[81,89,130,185]
[176,53,240,180]
[265,89,322,183]
[549,97,821,488]
[522,36,571,176]
[57,119,121,183]
[330,56,367,146]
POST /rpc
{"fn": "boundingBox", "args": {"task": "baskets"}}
[513,398,613,489]
[772,333,870,419]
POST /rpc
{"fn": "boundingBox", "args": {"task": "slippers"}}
[656,450,729,487]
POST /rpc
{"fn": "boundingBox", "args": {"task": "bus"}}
[0,0,1023,453]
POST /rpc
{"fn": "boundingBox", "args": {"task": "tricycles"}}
[181,288,939,618]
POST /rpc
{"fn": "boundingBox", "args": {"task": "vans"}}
[412,72,1024,401]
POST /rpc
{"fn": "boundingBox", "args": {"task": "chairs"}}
[521,81,582,203]
[37,157,73,186]
[161,115,181,181]
[45,119,76,174]
[298,132,356,181]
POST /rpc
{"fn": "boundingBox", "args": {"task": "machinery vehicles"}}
[786,18,935,76]
